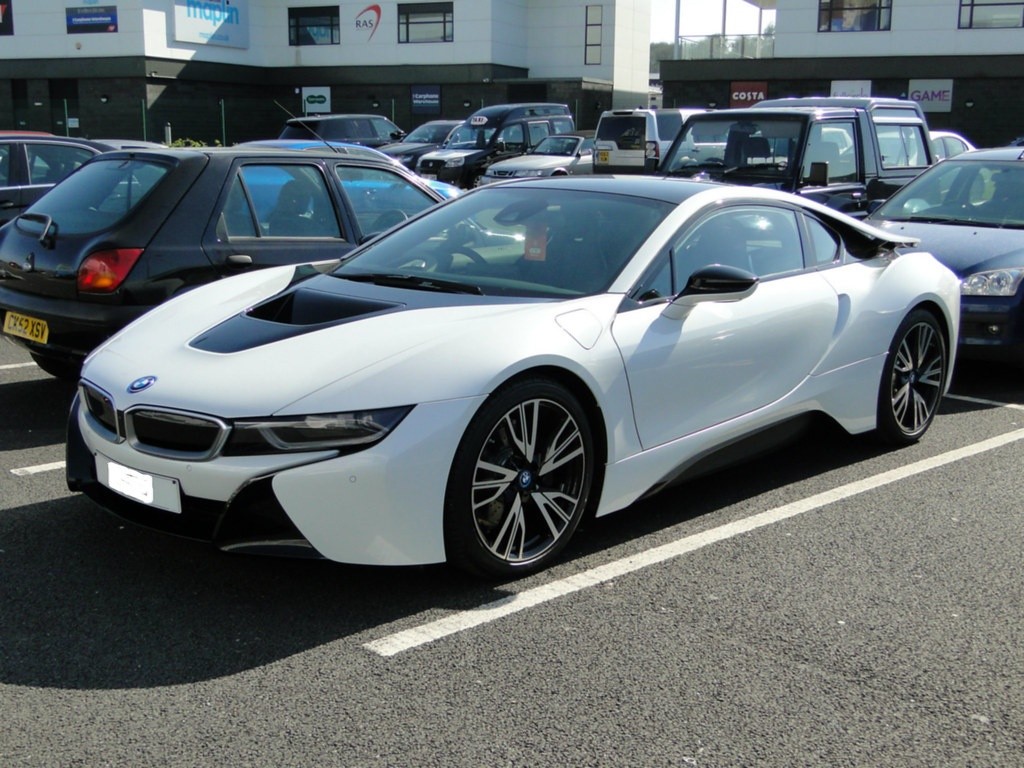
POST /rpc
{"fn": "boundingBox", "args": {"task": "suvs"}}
[655,95,936,218]
[589,107,727,172]
[280,113,408,147]
[1,146,448,378]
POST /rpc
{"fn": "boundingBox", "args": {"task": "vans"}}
[416,102,576,189]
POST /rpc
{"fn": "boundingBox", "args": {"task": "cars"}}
[860,146,1022,360]
[223,139,463,233]
[58,175,967,585]
[1,134,118,227]
[839,129,976,170]
[376,118,465,167]
[480,133,594,183]
